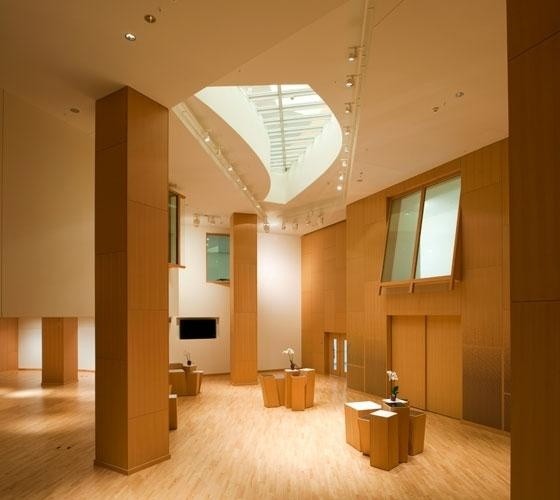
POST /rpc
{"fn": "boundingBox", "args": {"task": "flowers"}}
[383,369,401,395]
[281,347,296,365]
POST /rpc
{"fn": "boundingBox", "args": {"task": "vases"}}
[391,395,396,401]
[291,363,294,370]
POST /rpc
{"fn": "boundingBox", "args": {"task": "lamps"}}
[335,43,358,137]
[194,215,327,233]
